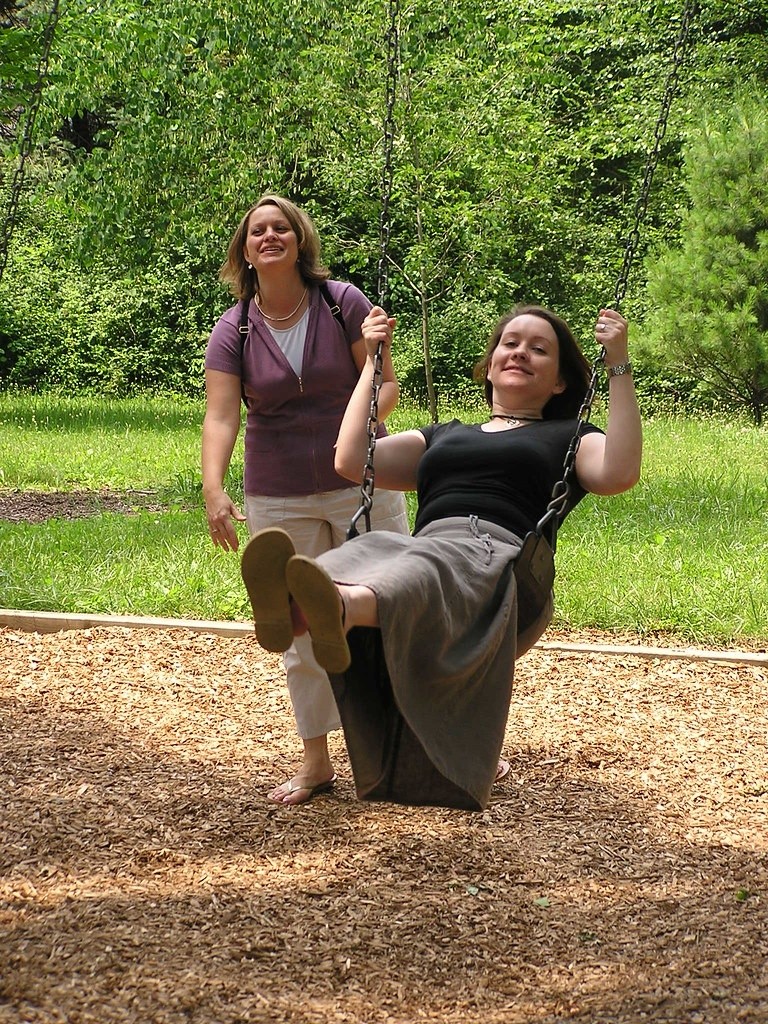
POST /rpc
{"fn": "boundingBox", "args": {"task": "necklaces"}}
[254,286,309,321]
[488,413,544,424]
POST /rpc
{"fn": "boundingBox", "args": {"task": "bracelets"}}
[605,361,633,380]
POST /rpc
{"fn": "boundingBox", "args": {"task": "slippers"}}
[241,527,296,653]
[284,554,350,672]
[267,774,337,803]
[493,760,511,782]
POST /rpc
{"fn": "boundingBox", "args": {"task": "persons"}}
[201,195,510,808]
[239,305,642,671]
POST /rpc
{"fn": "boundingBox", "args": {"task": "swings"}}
[341,0,695,640]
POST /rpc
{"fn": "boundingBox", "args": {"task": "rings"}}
[601,323,606,333]
[211,529,217,533]
